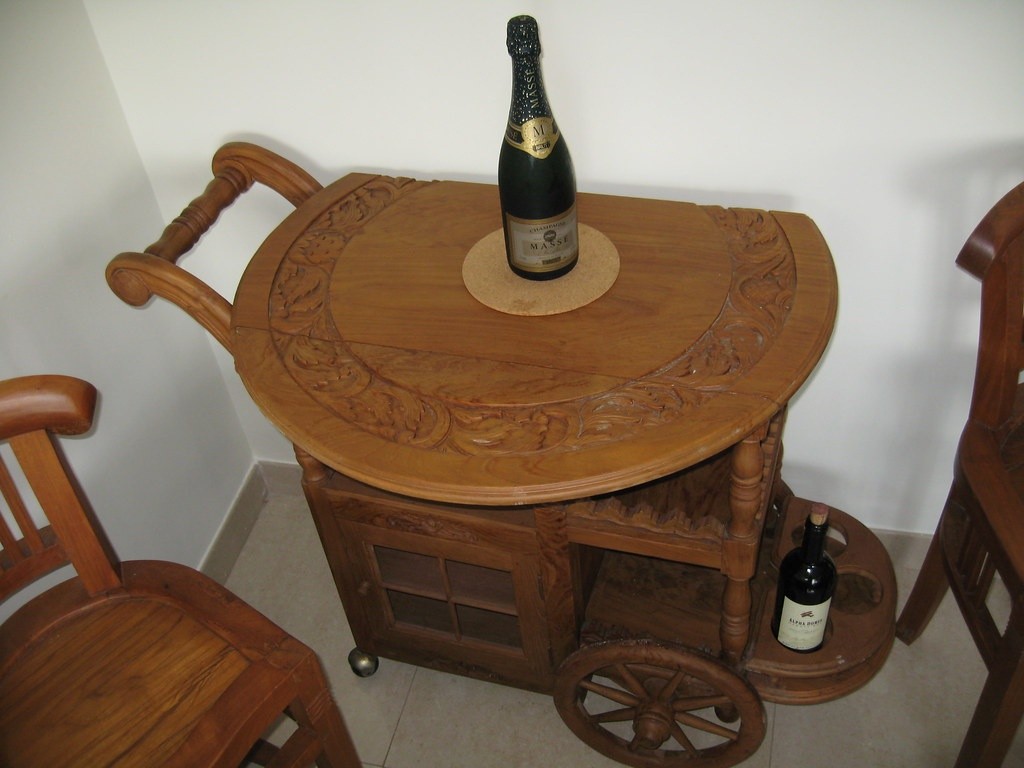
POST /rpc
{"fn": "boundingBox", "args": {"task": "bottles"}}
[772,502,838,654]
[498,14,580,280]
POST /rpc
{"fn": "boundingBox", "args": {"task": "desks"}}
[106,140,897,768]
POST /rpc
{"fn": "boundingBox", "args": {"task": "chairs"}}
[893,181,1024,768]
[0,375,360,768]
